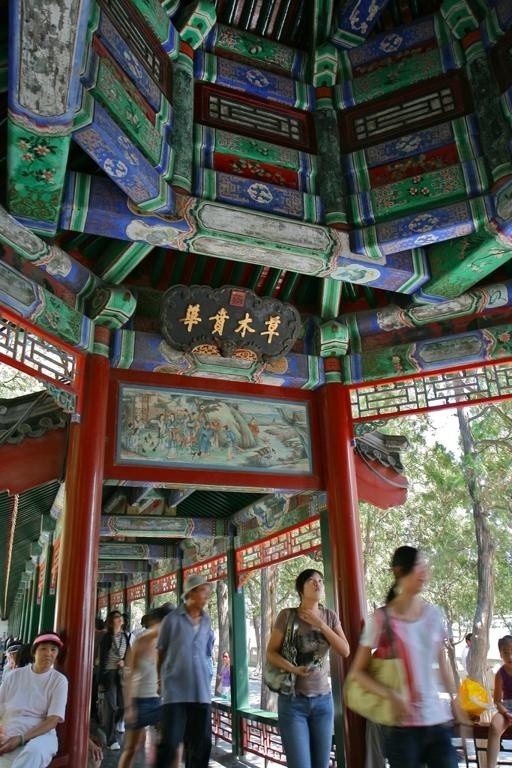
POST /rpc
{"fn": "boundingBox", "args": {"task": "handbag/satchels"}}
[263,607,300,696]
[342,606,407,726]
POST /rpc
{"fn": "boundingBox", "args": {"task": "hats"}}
[180,575,212,599]
[33,632,64,649]
[153,601,177,617]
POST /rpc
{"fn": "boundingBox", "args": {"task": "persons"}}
[88,602,177,768]
[0,631,69,768]
[153,574,215,768]
[217,651,231,694]
[351,546,464,768]
[267,569,350,768]
[466,633,512,767]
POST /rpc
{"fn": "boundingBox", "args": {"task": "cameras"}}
[305,659,320,674]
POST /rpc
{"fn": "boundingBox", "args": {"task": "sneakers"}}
[106,741,121,751]
[116,720,125,733]
